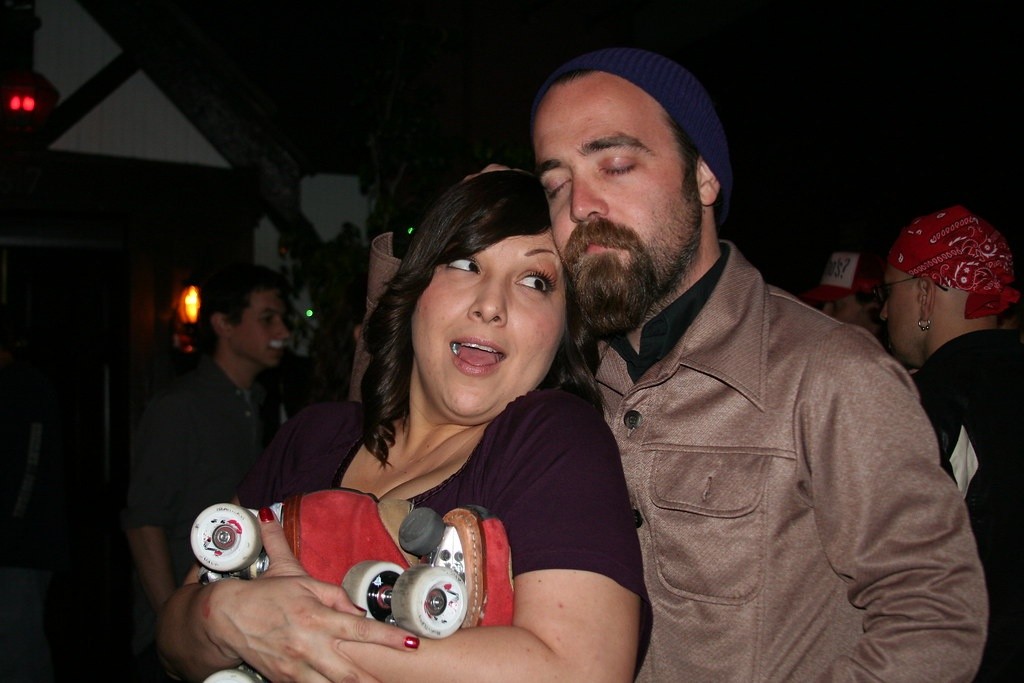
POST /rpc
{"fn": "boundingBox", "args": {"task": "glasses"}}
[872,275,949,305]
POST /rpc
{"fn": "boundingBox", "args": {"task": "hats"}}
[529,45,732,229]
[800,250,885,303]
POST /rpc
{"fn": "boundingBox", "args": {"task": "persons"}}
[159,171,649,683]
[802,251,887,339]
[0,304,65,683]
[120,264,290,683]
[871,205,1024,683]
[351,46,989,683]
